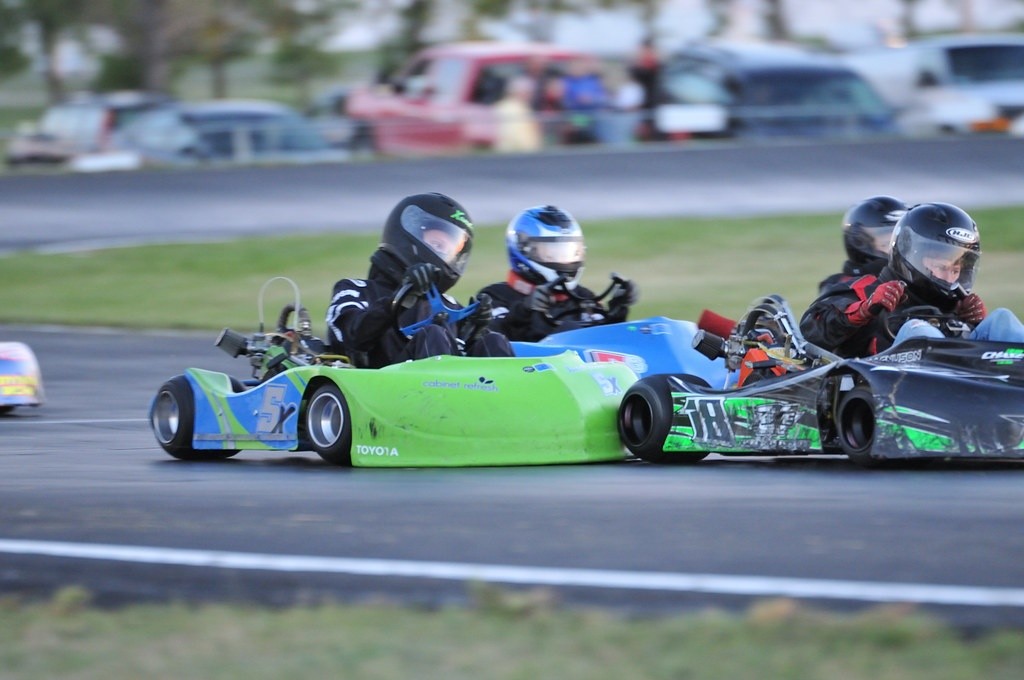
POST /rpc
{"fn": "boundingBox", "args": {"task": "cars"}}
[6,88,338,171]
[651,40,902,141]
[871,29,1024,139]
[343,38,693,156]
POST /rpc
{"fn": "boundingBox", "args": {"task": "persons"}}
[817,195,910,292]
[325,192,516,368]
[494,36,670,151]
[477,205,637,342]
[799,203,1023,358]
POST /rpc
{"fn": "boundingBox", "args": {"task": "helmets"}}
[505,202,585,294]
[369,192,474,301]
[888,203,982,309]
[840,196,910,278]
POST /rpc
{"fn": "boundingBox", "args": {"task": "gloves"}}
[607,271,638,311]
[521,284,552,315]
[953,294,987,327]
[862,278,904,318]
[465,292,493,327]
[398,261,441,296]
